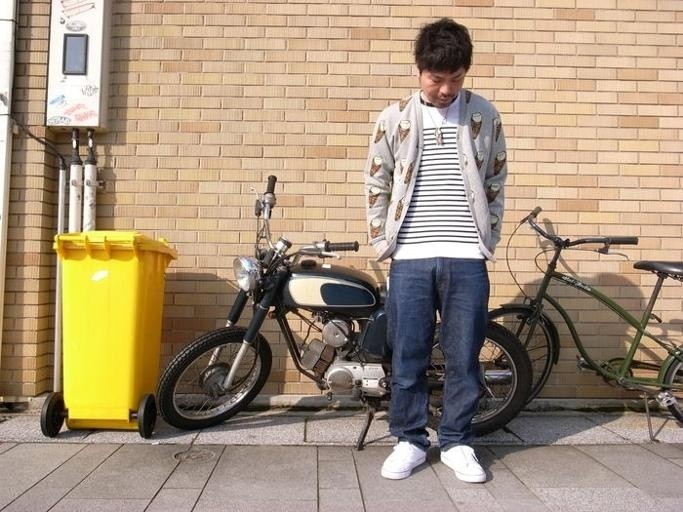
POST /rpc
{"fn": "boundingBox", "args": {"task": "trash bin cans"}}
[38,228,179,440]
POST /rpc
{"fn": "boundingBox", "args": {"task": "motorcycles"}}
[157,176,533,451]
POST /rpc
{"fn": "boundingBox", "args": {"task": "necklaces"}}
[420,88,450,146]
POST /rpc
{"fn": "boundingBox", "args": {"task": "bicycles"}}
[487,206,683,442]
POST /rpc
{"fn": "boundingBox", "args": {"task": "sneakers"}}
[440,444,486,484]
[381,441,428,480]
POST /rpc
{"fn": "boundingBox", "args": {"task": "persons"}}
[366,17,508,484]
